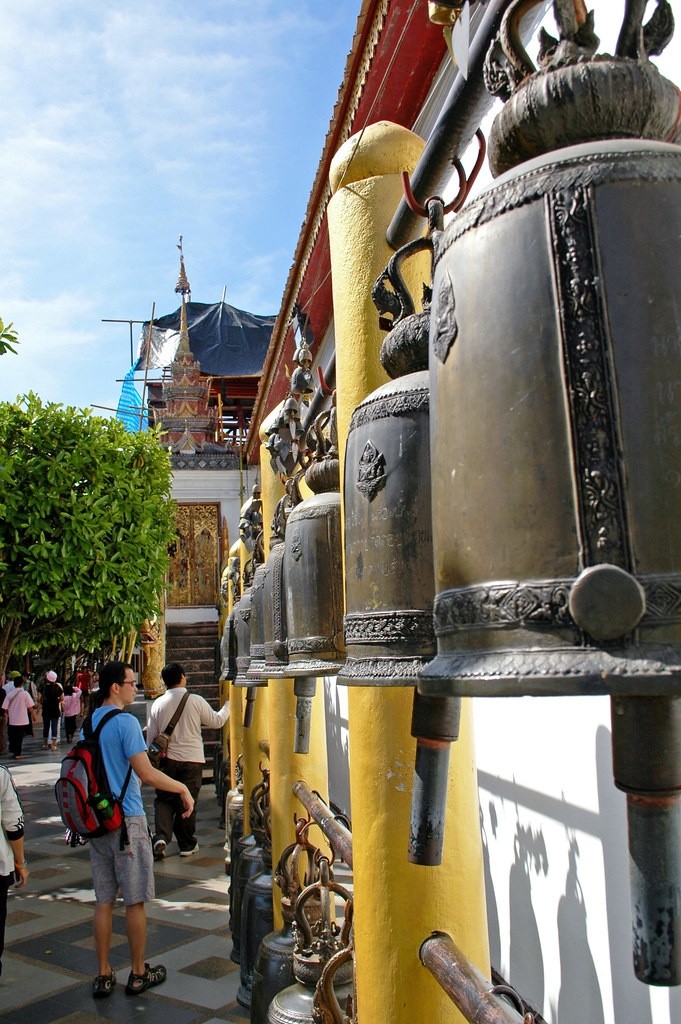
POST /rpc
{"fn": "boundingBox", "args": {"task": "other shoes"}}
[180,843,199,856]
[154,839,166,858]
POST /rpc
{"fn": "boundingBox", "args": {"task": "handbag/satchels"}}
[147,733,170,770]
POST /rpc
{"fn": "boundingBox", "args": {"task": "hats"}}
[10,671,21,678]
[47,671,57,682]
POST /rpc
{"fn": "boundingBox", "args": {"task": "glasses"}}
[113,680,136,688]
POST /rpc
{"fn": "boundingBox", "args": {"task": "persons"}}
[0,763,30,977]
[78,660,195,998]
[145,662,231,858]
[0,666,100,759]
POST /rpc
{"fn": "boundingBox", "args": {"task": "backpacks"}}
[55,707,133,839]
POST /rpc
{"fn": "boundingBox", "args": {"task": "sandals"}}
[92,970,116,998]
[125,963,166,996]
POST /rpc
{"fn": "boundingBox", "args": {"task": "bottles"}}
[94,793,114,820]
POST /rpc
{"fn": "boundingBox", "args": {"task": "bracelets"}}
[14,859,29,870]
